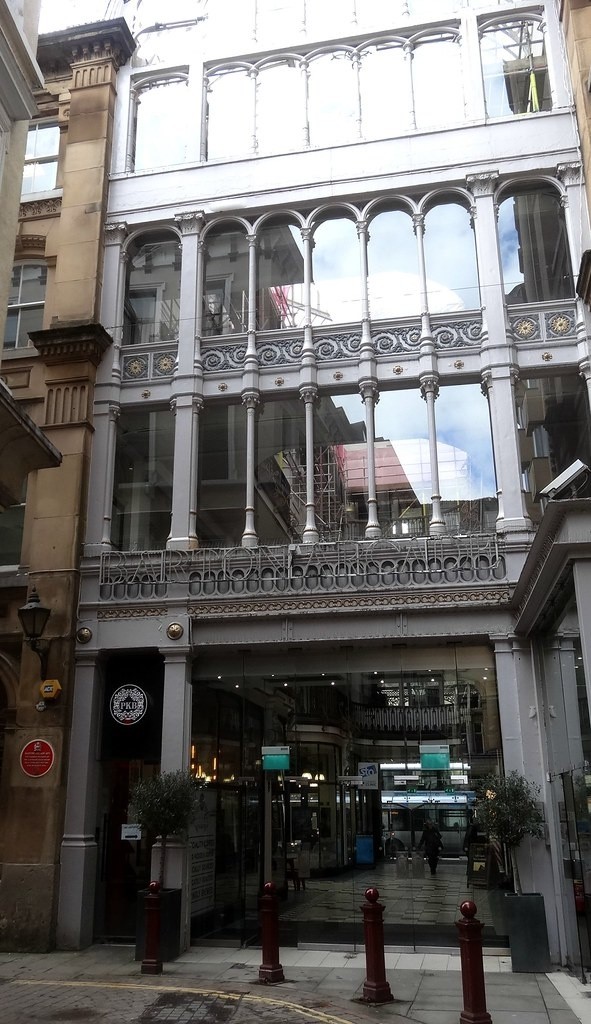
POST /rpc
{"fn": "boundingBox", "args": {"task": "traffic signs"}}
[120,823,142,840]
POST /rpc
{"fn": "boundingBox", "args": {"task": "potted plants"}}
[127,770,203,962]
[471,768,554,973]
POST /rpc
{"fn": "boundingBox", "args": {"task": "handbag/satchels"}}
[431,831,444,849]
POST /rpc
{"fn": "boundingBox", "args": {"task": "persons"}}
[463,825,475,875]
[418,817,442,875]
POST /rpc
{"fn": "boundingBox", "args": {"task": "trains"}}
[243,789,476,860]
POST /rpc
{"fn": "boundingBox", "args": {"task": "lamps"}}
[15,584,53,682]
